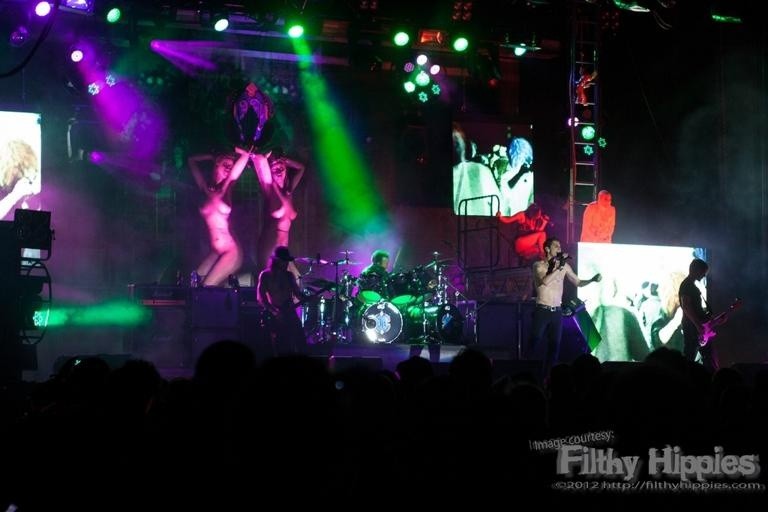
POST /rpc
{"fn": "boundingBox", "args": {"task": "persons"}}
[0,140,42,221]
[527,237,602,361]
[453,131,534,217]
[581,190,616,243]
[680,258,729,375]
[251,148,305,272]
[256,245,308,352]
[574,51,598,104]
[361,249,393,299]
[2,339,767,509]
[495,203,550,267]
[187,144,248,286]
[407,344,440,361]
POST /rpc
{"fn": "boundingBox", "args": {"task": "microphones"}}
[558,251,572,260]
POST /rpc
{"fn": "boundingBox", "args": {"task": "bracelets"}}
[557,267,564,272]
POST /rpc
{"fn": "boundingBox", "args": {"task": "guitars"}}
[696,296,745,347]
[257,285,332,328]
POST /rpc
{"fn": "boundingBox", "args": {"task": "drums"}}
[304,298,336,326]
[353,290,382,313]
[403,304,464,345]
[331,294,355,329]
[415,292,439,305]
[357,298,403,344]
[391,295,416,316]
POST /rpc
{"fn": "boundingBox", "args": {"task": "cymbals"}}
[294,258,327,266]
[330,259,360,265]
[339,251,354,254]
[425,258,454,269]
[306,278,343,291]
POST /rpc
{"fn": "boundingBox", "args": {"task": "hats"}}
[268,247,294,260]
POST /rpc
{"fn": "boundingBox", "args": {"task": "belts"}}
[535,303,562,312]
